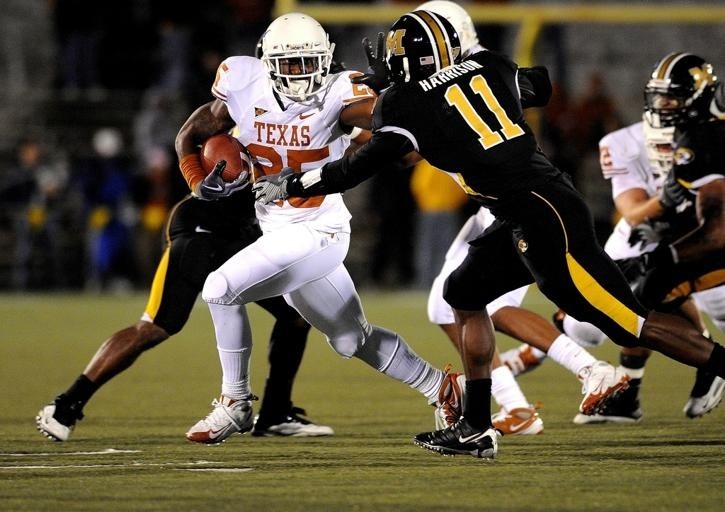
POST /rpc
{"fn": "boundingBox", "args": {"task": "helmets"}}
[384,0,479,84]
[256,11,336,103]
[641,49,719,134]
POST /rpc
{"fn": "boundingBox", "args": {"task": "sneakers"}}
[433,342,550,435]
[186,396,253,443]
[34,394,77,444]
[412,418,500,460]
[682,342,724,418]
[253,408,336,439]
[573,357,644,428]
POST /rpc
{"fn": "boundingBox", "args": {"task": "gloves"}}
[662,174,686,209]
[198,157,250,203]
[350,32,392,96]
[614,252,652,285]
[253,167,295,207]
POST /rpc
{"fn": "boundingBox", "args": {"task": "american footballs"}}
[200,133,250,182]
